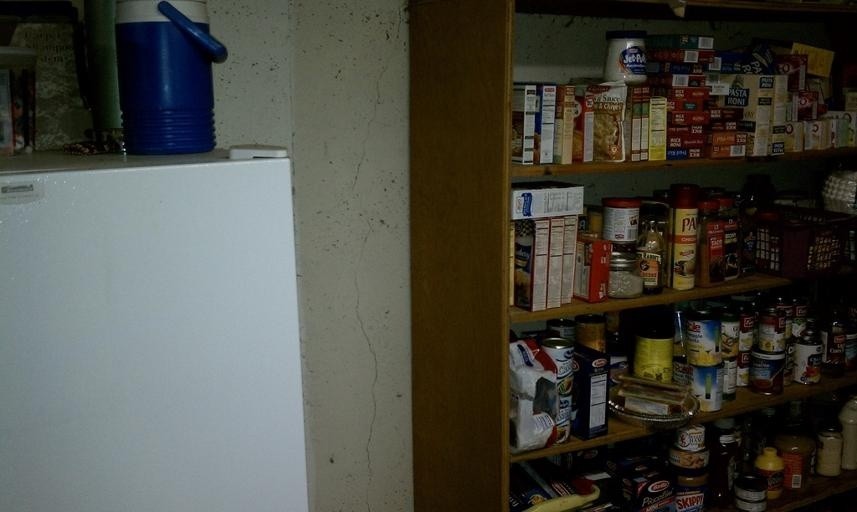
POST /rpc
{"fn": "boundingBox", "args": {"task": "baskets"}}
[748,206,854,282]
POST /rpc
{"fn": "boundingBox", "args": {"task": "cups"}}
[114,1,228,156]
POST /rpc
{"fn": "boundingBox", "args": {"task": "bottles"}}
[707,393,857,511]
[798,312,856,386]
[607,252,642,298]
[634,217,664,294]
[696,183,744,287]
[604,29,650,85]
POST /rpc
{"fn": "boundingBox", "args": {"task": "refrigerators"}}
[1,140,308,509]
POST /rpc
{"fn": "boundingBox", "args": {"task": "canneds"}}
[524,311,620,444]
[720,293,808,401]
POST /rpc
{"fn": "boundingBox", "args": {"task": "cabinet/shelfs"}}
[408,0,855,511]
